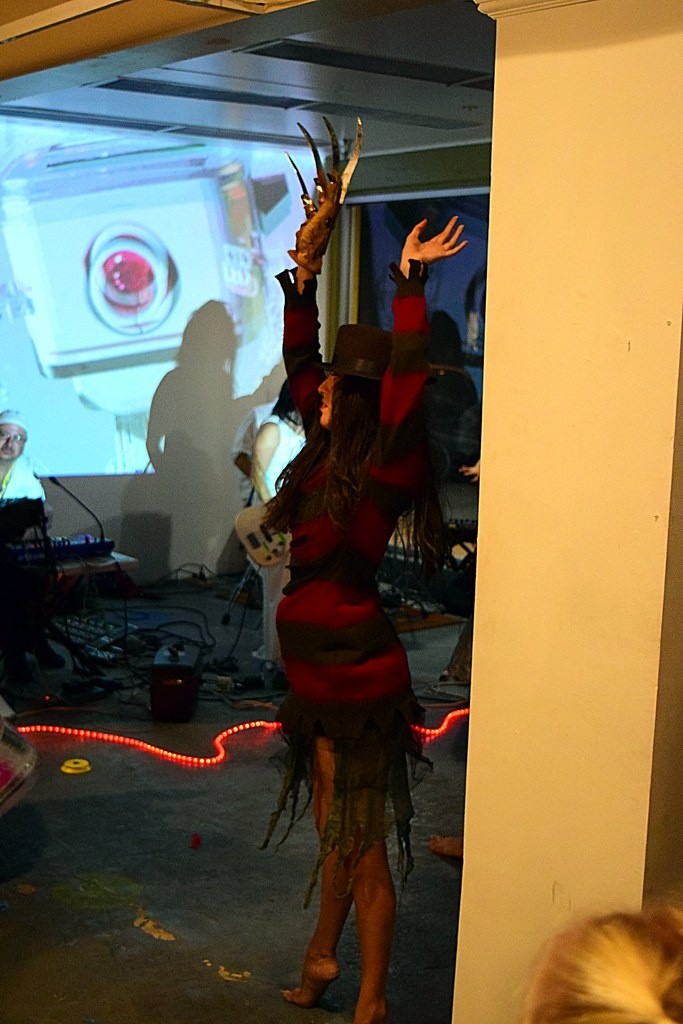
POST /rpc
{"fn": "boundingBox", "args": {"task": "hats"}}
[316,324,392,380]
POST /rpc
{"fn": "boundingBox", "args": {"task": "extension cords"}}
[197,579,212,588]
[43,677,108,708]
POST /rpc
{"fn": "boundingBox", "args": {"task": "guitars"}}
[234,498,292,568]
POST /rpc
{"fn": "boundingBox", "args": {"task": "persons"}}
[439,455,480,688]
[233,373,307,664]
[0,409,46,502]
[261,116,469,1024]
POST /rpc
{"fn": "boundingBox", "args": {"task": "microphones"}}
[49,476,105,541]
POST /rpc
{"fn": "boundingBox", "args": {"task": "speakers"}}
[150,643,204,724]
